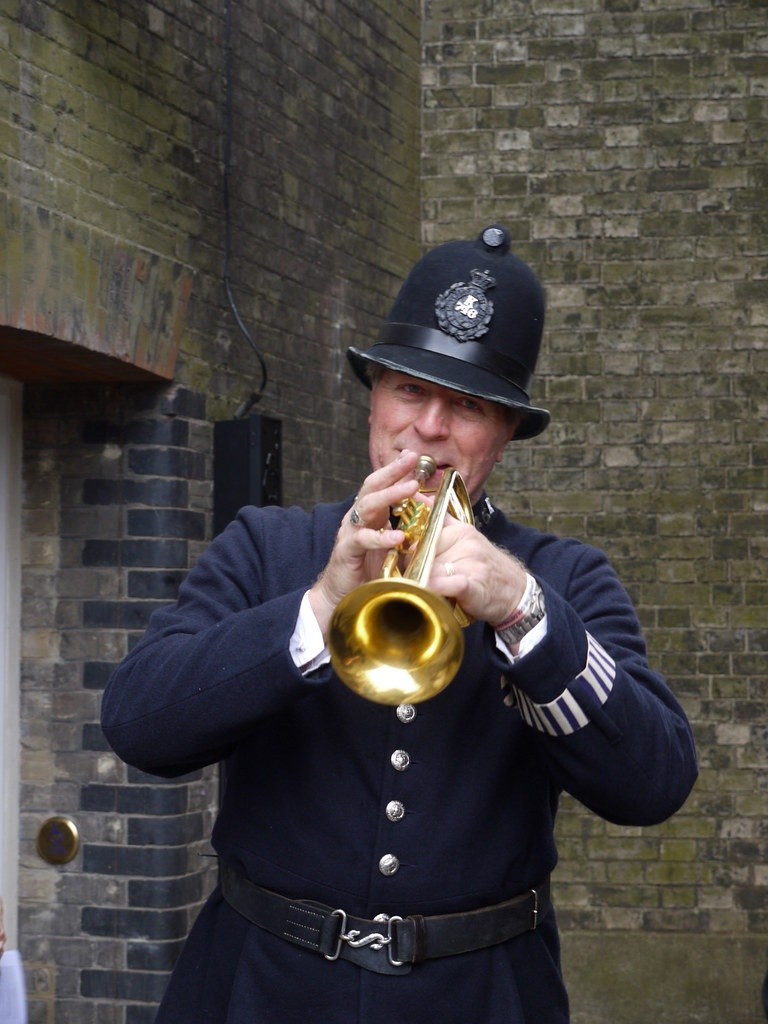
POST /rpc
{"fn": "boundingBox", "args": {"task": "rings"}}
[350,508,367,527]
[355,496,358,500]
[444,562,455,576]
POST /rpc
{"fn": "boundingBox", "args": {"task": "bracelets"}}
[495,572,536,632]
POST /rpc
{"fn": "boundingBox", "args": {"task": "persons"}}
[100,224,697,1024]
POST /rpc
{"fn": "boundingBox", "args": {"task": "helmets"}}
[347,224,550,441]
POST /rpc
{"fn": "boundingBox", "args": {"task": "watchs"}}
[499,589,547,644]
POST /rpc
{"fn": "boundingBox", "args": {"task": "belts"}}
[218,858,549,976]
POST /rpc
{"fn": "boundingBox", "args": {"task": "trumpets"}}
[326,451,477,709]
